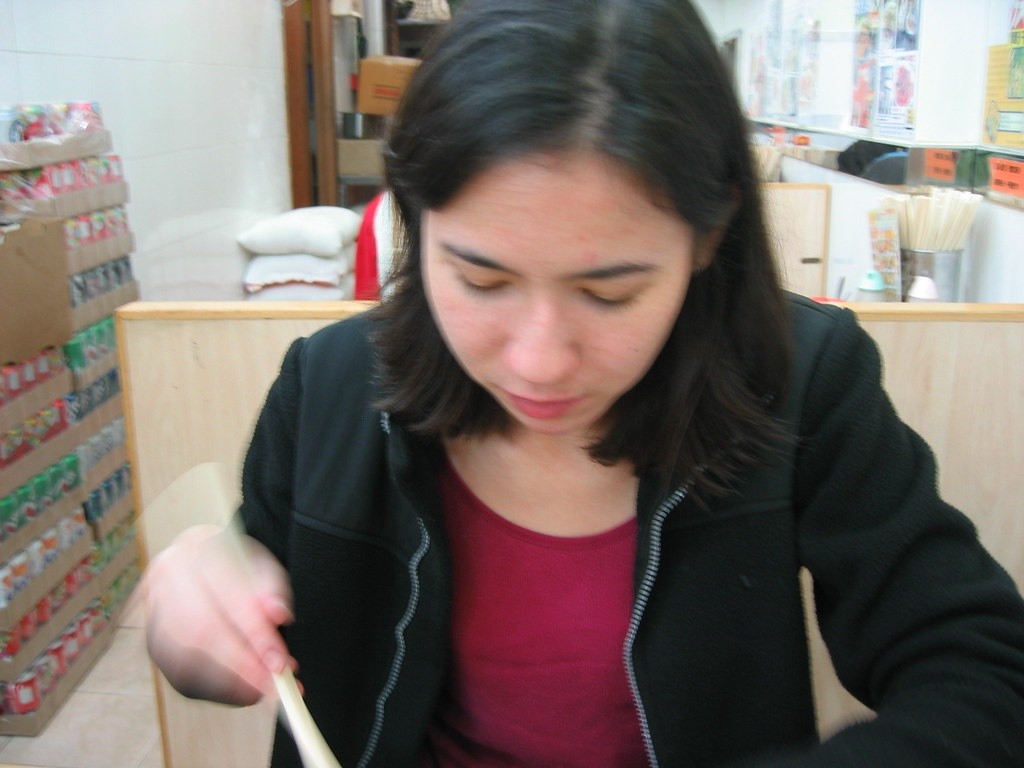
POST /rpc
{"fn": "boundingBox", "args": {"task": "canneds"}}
[1,100,141,714]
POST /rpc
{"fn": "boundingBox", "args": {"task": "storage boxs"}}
[0,216,74,364]
[360,55,423,118]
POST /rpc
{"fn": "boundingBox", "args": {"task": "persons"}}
[135,0,1024,768]
